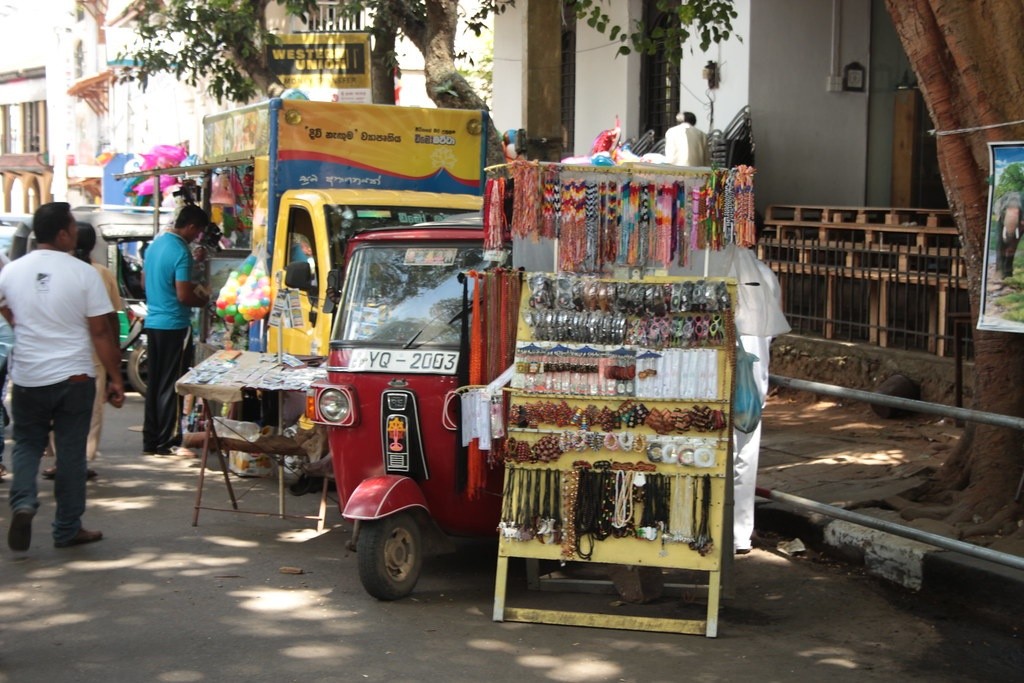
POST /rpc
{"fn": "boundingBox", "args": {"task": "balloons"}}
[124,144,208,213]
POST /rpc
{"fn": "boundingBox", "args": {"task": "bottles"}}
[182,390,279,443]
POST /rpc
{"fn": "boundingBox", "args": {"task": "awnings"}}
[66,70,109,113]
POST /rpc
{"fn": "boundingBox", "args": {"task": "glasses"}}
[525,273,731,350]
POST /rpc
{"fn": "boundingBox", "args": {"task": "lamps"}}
[702,60,717,87]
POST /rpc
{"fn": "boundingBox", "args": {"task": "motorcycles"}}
[305,212,525,599]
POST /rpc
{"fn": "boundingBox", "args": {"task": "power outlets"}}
[825,76,842,92]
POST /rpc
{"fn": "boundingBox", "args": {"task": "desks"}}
[173,351,336,533]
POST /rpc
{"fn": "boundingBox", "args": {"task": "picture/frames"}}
[844,62,866,92]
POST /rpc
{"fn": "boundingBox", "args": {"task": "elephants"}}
[993,191,1024,279]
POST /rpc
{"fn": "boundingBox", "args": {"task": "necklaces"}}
[497,468,713,561]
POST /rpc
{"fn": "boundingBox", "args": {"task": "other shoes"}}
[42,466,58,477]
[0,464,14,480]
[8,509,33,551]
[86,467,97,478]
[54,528,102,546]
[143,443,172,455]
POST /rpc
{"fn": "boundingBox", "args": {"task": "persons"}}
[141,205,210,456]
[0,203,124,550]
[733,245,783,554]
[665,112,709,166]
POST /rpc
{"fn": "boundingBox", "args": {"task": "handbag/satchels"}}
[734,323,762,433]
[728,251,792,338]
